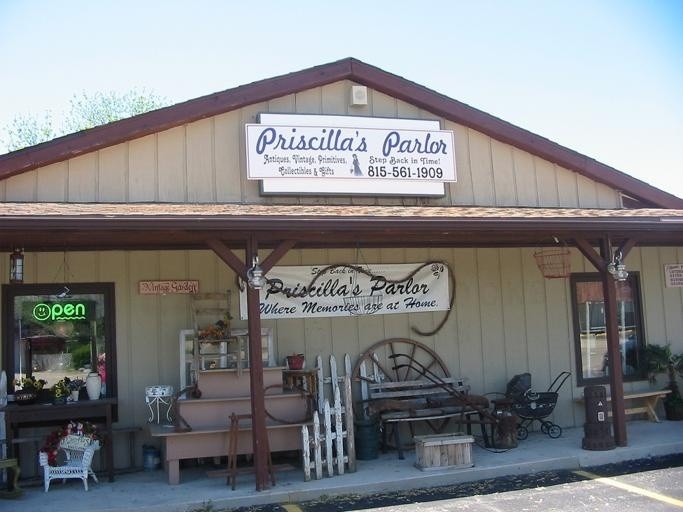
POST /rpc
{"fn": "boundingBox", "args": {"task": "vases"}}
[87,373,102,399]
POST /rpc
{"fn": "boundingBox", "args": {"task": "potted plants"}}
[642,344,683,420]
[69,376,86,401]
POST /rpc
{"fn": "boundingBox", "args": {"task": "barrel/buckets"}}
[142,443,162,471]
[353,418,380,459]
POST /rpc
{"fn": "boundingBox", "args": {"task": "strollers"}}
[505,371,570,441]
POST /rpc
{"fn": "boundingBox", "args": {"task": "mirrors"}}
[571,270,647,386]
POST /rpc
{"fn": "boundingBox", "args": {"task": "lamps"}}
[608,253,628,281]
[247,256,267,290]
[10,245,24,284]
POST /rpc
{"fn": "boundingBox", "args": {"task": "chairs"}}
[192,289,243,382]
[1,439,25,499]
[40,423,103,492]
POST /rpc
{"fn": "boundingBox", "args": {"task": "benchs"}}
[575,390,671,423]
[368,377,490,459]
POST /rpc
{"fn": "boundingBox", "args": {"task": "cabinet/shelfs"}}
[0,282,118,422]
[4,397,116,482]
[148,369,313,486]
